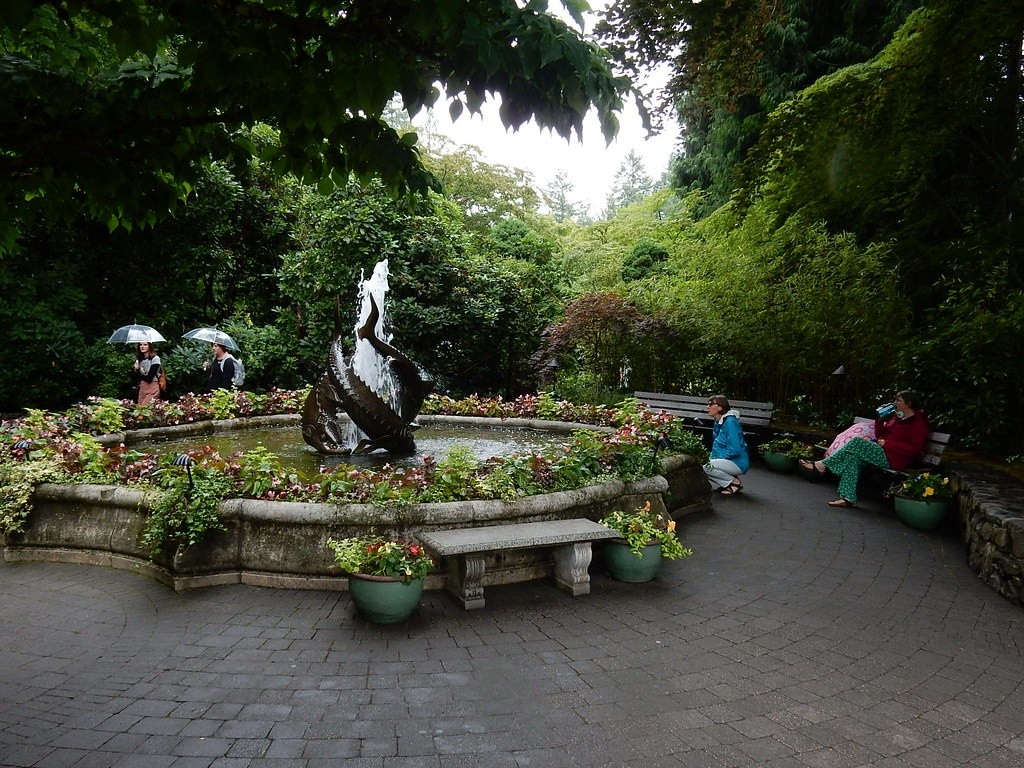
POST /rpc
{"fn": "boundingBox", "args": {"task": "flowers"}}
[756,432,828,459]
[326,525,435,588]
[881,466,954,505]
[598,501,693,562]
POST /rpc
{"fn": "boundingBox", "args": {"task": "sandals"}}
[799,459,826,476]
[720,481,743,497]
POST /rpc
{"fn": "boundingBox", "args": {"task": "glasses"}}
[706,402,717,405]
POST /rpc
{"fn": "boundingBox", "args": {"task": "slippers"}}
[828,499,858,508]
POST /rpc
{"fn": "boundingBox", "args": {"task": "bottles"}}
[874,402,904,418]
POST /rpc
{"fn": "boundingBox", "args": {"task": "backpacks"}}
[151,355,166,392]
[220,356,245,386]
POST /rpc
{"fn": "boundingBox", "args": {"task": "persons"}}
[202,342,236,393]
[132,341,163,407]
[798,390,934,508]
[702,395,750,496]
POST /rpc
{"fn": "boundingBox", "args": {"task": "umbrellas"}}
[105,323,168,370]
[181,328,241,371]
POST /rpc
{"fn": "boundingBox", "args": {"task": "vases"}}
[346,571,424,623]
[797,456,824,484]
[894,493,951,531]
[763,449,797,473]
[601,537,663,583]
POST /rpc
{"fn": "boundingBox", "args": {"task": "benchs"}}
[634,391,774,462]
[416,516,625,610]
[816,415,952,518]
